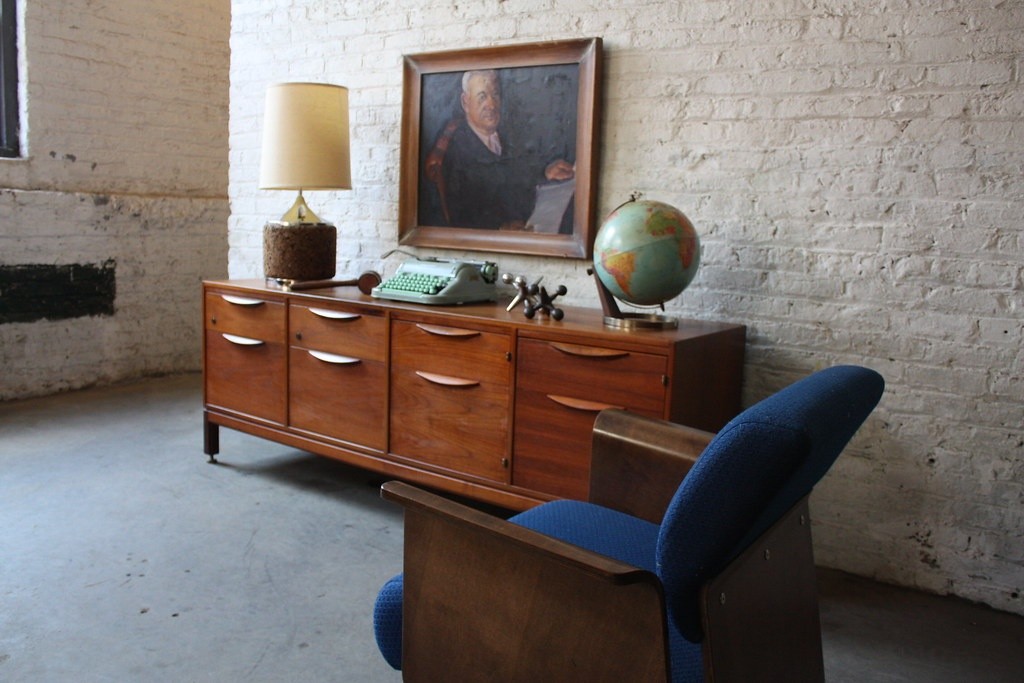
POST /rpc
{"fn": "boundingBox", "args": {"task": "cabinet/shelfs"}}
[198,278,747,520]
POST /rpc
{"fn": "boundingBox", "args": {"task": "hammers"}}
[290,270,381,296]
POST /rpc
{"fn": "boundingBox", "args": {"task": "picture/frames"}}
[399,36,602,261]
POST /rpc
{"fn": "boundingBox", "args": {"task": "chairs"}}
[371,362,885,683]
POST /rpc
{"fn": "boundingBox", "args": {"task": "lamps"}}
[256,81,353,278]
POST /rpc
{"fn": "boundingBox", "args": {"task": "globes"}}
[587,192,701,331]
